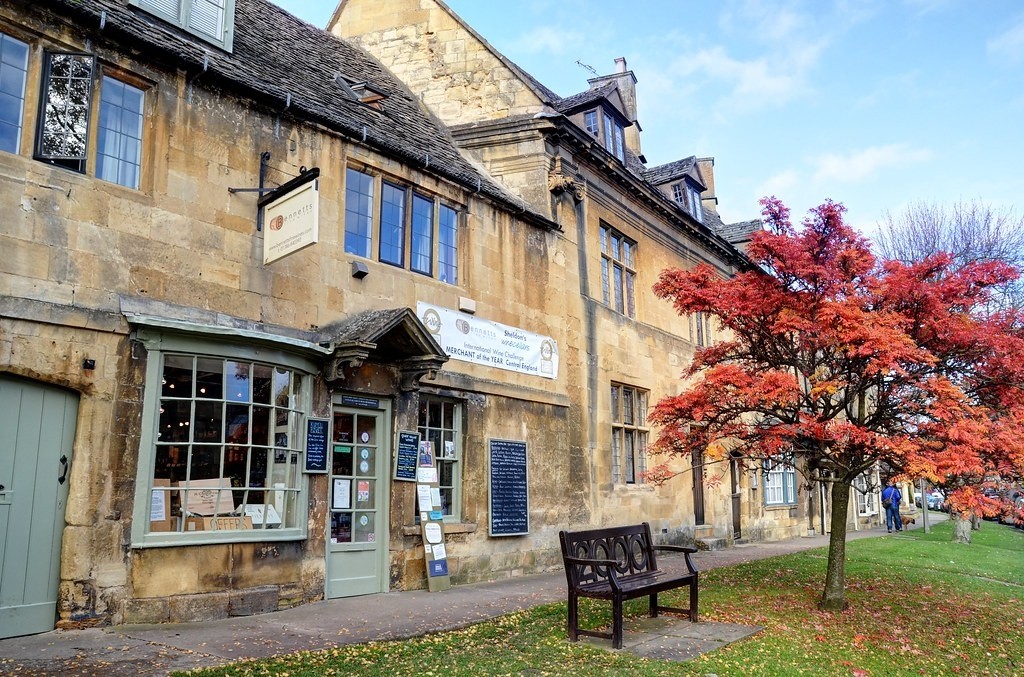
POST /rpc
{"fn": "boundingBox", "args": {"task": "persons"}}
[881,479,903,532]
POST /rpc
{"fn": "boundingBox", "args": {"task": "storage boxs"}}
[184,516,253,531]
[150,478,171,532]
[171,515,178,531]
[234,503,282,529]
[170,477,235,516]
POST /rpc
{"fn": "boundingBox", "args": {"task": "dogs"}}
[892,515,915,531]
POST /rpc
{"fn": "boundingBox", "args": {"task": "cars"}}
[915,487,1024,530]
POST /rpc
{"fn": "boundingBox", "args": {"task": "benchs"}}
[559,521,699,649]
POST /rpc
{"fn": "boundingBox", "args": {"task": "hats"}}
[887,481,894,487]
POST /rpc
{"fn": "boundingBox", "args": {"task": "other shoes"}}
[899,528,903,531]
[888,529,892,533]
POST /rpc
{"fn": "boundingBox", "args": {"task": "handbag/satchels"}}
[883,498,892,508]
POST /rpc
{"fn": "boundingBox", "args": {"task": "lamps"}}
[352,260,368,278]
